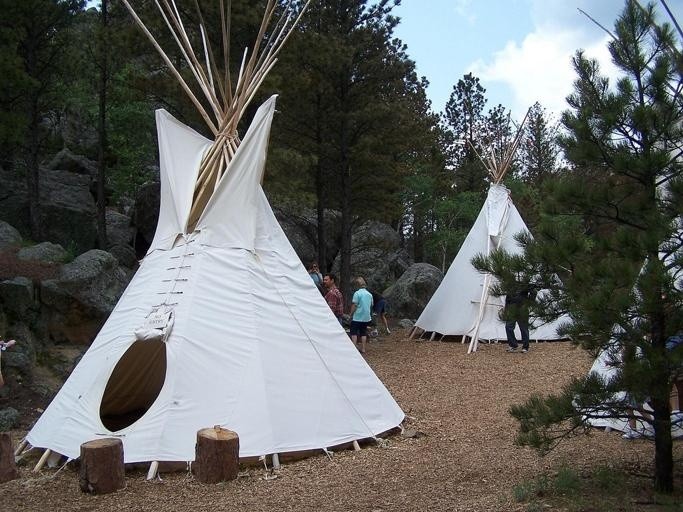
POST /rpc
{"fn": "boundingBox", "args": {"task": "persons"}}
[307,261,391,353]
[505,272,537,354]
[619,292,683,442]
[0,335,5,388]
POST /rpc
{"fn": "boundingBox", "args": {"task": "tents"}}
[571,212,683,442]
[408,182,593,344]
[16,94,407,478]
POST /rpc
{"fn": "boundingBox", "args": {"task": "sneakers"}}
[521,348,528,353]
[372,331,378,335]
[507,347,517,352]
[385,329,392,334]
